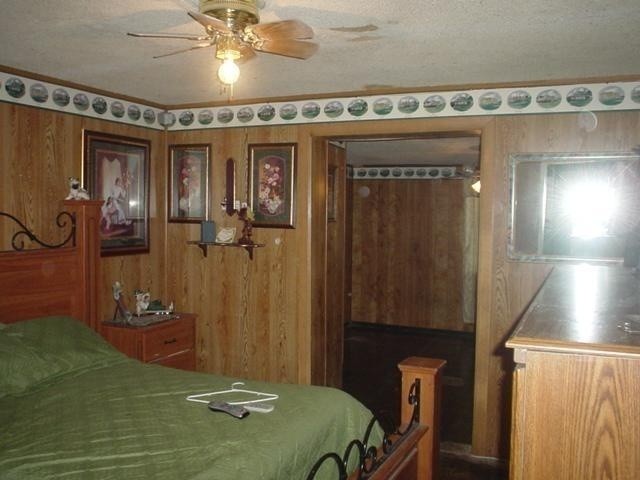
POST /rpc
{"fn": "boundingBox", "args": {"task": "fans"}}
[125,0,320,60]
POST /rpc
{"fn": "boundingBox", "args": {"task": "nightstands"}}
[101,311,199,372]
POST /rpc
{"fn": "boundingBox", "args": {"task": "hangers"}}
[185,381,279,405]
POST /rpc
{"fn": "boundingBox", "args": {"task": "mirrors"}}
[502,149,640,266]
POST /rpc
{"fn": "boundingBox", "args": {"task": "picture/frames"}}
[327,164,340,223]
[166,140,299,230]
[79,128,151,258]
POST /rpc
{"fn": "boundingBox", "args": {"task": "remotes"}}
[207,400,249,419]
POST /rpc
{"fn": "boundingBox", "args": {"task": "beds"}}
[0,210,447,480]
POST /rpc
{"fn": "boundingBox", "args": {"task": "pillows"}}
[0,315,135,399]
